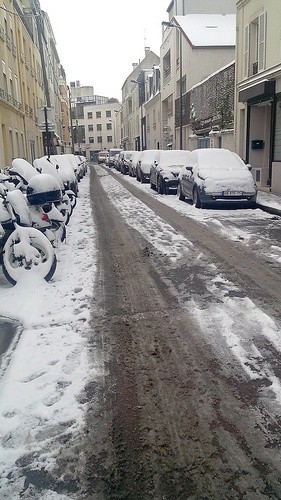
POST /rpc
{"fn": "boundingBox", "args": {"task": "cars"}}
[150,150,191,194]
[137,150,159,183]
[91,148,140,177]
[64,154,87,180]
[177,148,258,210]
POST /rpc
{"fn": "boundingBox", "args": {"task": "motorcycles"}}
[0,156,76,286]
